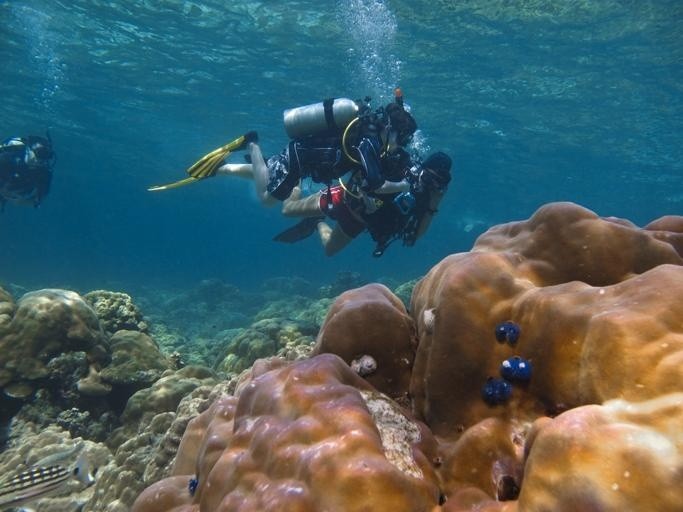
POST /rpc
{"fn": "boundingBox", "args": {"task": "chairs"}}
[312,216,325,230]
[231,131,257,152]
[204,159,225,178]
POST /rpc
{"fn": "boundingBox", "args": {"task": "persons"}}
[198,103,417,207]
[281,153,453,258]
[0,136,54,213]
